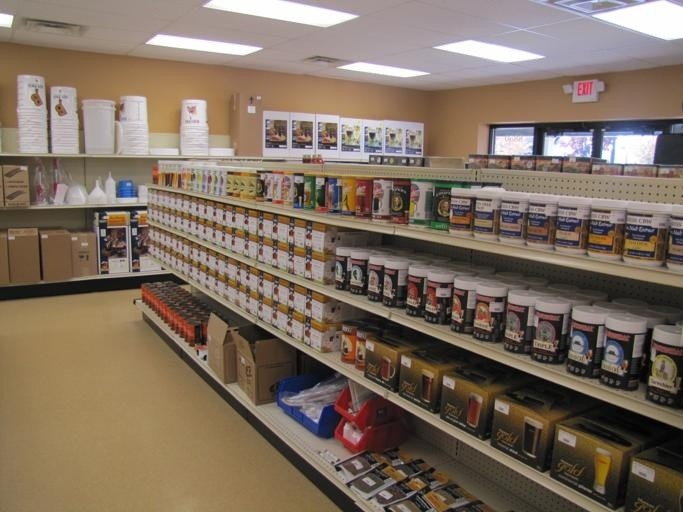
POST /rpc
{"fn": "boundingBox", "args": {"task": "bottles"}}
[104,171,116,202]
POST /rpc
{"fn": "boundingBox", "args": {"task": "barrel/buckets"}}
[81,100,115,153]
[180,99,208,158]
[117,94,149,155]
[50,86,80,153]
[17,74,49,153]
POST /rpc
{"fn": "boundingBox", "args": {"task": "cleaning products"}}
[106,169,116,202]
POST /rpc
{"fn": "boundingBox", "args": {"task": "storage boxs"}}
[3,164,30,209]
[1,225,98,286]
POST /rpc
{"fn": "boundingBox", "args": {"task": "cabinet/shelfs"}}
[132,158,682,511]
[0,153,369,287]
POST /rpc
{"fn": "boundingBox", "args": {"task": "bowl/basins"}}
[118,180,134,199]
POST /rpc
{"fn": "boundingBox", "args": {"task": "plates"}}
[269,134,337,145]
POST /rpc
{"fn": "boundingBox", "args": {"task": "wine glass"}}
[346,127,416,146]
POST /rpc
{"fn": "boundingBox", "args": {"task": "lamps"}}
[562,78,606,103]
